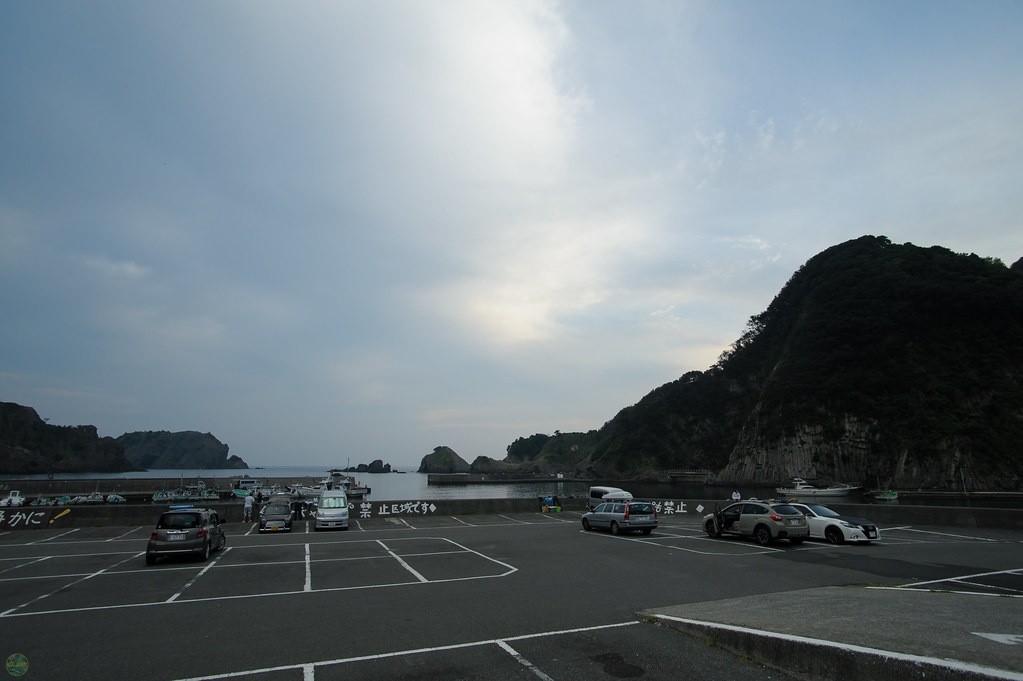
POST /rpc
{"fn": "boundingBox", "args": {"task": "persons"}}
[241,491,255,522]
[537,495,563,515]
[4,489,122,520]
[353,480,364,489]
[250,479,316,522]
[732,489,741,502]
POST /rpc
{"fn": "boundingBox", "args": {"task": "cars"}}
[314,489,352,532]
[701,500,810,543]
[781,501,882,546]
[266,493,293,511]
[258,504,295,533]
[792,477,803,485]
[145,508,227,560]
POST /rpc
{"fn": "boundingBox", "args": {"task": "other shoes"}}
[242,519,253,524]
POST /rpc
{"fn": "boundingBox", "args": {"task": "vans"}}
[589,486,633,510]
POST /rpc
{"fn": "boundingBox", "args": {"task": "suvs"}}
[581,502,659,536]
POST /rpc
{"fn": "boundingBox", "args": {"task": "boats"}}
[0,488,128,507]
[873,490,897,502]
[775,481,851,498]
[149,479,221,506]
[215,468,373,501]
